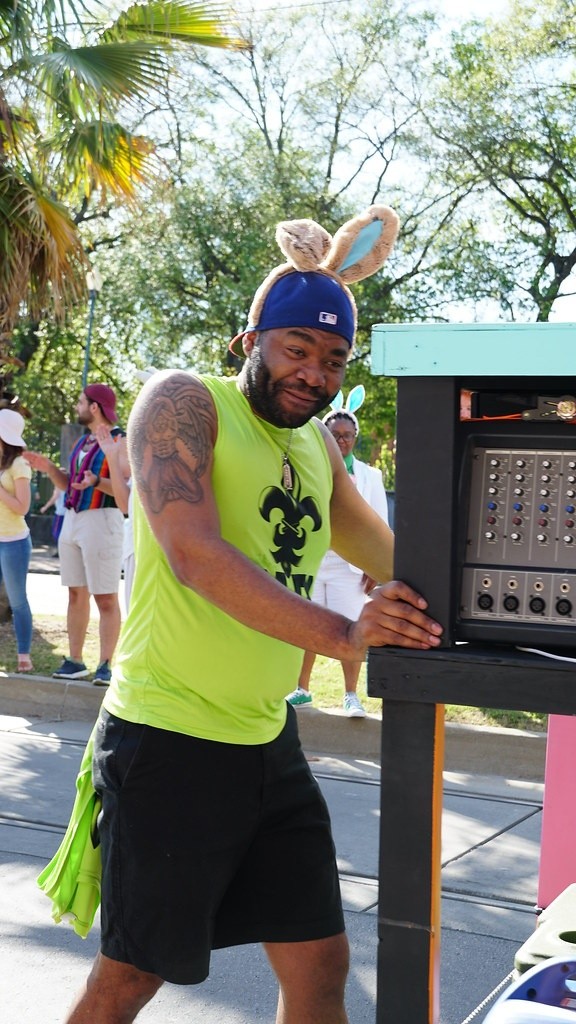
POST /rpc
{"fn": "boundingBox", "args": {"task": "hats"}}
[84,384,118,422]
[229,272,354,361]
[0,409,27,447]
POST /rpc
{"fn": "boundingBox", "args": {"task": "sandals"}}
[16,660,34,674]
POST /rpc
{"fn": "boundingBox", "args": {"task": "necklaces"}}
[262,429,295,491]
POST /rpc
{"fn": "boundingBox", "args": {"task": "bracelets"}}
[93,475,101,487]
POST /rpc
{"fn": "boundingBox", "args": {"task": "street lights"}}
[82,290,97,386]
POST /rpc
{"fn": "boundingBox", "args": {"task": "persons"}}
[0,408,34,671]
[40,467,67,558]
[283,413,388,717]
[95,422,134,616]
[22,384,131,686]
[63,271,444,1024]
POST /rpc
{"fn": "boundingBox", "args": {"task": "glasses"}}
[332,431,355,442]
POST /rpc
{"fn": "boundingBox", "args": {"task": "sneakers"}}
[52,655,89,679]
[285,687,312,707]
[344,694,365,717]
[92,659,112,685]
